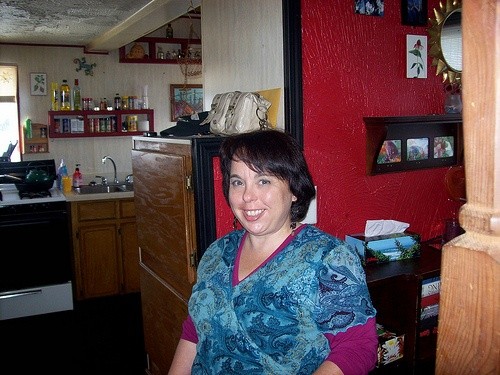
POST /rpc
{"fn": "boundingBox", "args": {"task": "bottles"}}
[82,98,108,111]
[157,47,182,59]
[57,158,68,190]
[166,23,173,38]
[54,116,117,133]
[122,95,148,111]
[51,82,59,111]
[73,164,81,189]
[26,118,32,139]
[60,79,71,111]
[73,79,81,111]
[114,93,121,110]
[40,128,46,138]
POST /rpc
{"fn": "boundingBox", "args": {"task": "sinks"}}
[75,186,123,194]
[122,184,135,192]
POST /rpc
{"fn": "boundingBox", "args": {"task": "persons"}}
[167,130,378,375]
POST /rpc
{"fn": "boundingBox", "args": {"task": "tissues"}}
[344,219,421,267]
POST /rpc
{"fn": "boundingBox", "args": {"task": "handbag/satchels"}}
[199,91,271,135]
[160,111,210,137]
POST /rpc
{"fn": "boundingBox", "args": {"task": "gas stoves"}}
[0,159,67,218]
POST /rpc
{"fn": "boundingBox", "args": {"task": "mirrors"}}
[426,0,463,89]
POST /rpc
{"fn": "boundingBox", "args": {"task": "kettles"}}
[26,161,54,192]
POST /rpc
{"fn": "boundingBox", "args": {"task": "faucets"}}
[101,155,119,184]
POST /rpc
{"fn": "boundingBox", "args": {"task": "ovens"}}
[0,210,77,322]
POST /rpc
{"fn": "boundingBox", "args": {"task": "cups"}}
[138,121,149,131]
[62,175,73,191]
[122,115,138,132]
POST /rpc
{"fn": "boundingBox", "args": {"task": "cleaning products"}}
[56,158,68,192]
[72,164,83,187]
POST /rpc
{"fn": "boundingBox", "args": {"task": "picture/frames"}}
[170,84,203,121]
[31,72,47,96]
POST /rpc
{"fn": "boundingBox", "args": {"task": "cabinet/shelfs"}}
[119,16,202,64]
[73,200,141,300]
[130,134,237,375]
[365,242,451,375]
[48,109,155,138]
[24,123,50,154]
